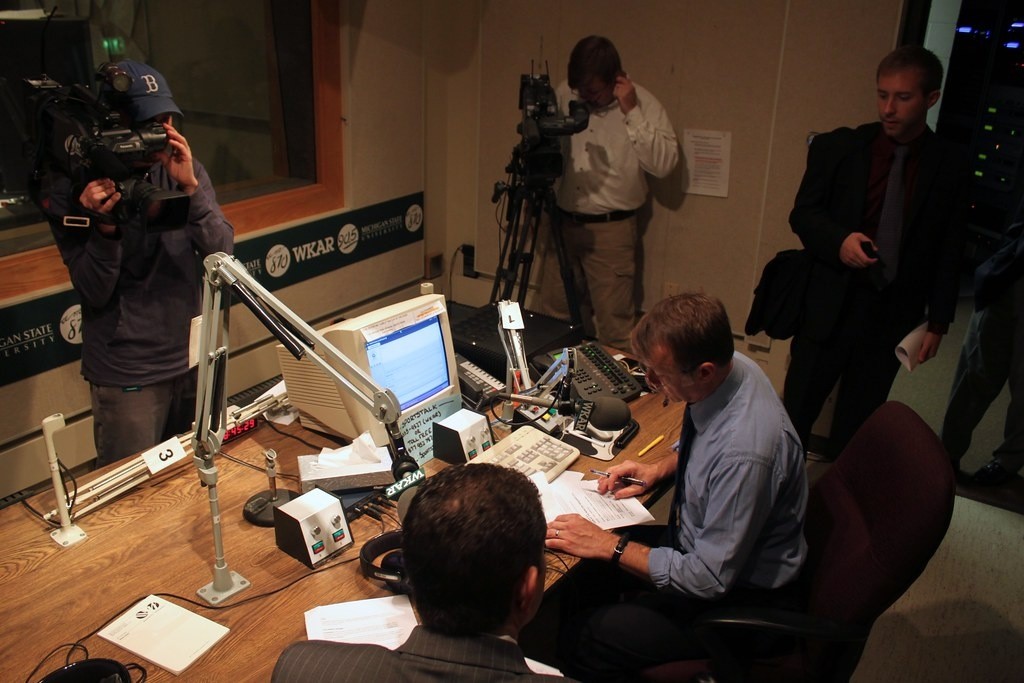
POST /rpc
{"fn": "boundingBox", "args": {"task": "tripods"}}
[484,137,585,345]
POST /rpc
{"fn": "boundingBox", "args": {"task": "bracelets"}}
[611,532,630,568]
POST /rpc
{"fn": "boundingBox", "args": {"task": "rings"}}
[555,530,560,536]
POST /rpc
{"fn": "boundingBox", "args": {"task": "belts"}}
[557,203,647,224]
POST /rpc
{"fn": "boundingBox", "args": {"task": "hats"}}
[102,57,185,125]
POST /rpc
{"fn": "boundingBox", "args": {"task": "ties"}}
[871,146,908,290]
[666,403,697,549]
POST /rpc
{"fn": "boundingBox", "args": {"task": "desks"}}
[0,335,687,683]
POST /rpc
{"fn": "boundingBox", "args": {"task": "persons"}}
[939,220,1024,488]
[522,290,808,683]
[47,57,234,470]
[271,462,581,683]
[550,36,678,355]
[783,46,973,461]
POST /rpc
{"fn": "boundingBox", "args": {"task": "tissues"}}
[296,429,398,494]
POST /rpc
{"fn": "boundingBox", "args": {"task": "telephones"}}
[454,352,505,410]
[528,340,643,403]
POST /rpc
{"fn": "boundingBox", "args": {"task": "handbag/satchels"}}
[744,249,812,340]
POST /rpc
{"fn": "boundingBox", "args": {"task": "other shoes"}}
[949,457,961,474]
[970,461,1018,487]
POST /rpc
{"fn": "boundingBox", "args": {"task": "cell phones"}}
[860,241,876,259]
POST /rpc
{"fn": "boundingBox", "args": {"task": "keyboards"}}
[465,425,584,484]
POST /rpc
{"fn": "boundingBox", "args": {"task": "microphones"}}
[90,145,130,182]
[388,422,420,482]
[499,393,632,432]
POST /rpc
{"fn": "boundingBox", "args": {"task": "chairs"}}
[636,400,957,683]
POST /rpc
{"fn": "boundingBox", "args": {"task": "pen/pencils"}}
[639,434,665,456]
[590,469,644,486]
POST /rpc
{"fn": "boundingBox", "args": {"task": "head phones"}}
[360,528,412,595]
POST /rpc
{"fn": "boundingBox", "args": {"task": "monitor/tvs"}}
[276,293,462,448]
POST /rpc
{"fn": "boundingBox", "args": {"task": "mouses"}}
[585,420,613,441]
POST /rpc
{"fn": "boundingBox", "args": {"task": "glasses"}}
[638,361,695,393]
[571,79,614,97]
